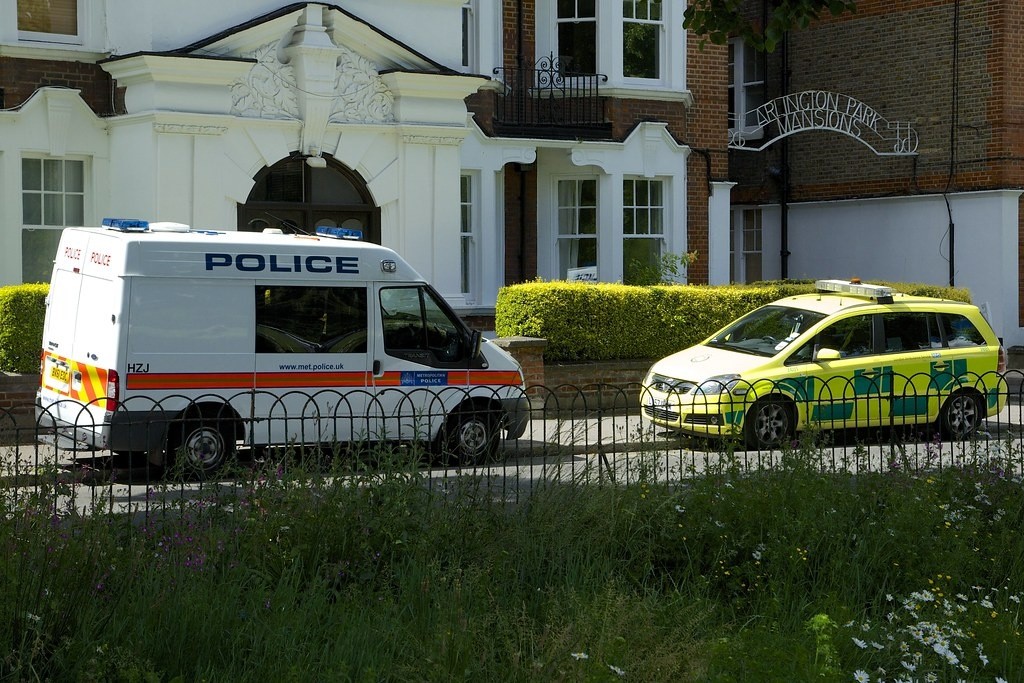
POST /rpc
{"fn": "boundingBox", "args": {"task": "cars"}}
[637,278,1010,451]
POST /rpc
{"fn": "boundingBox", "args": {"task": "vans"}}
[31,217,531,482]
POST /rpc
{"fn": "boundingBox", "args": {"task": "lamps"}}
[307,149,326,167]
